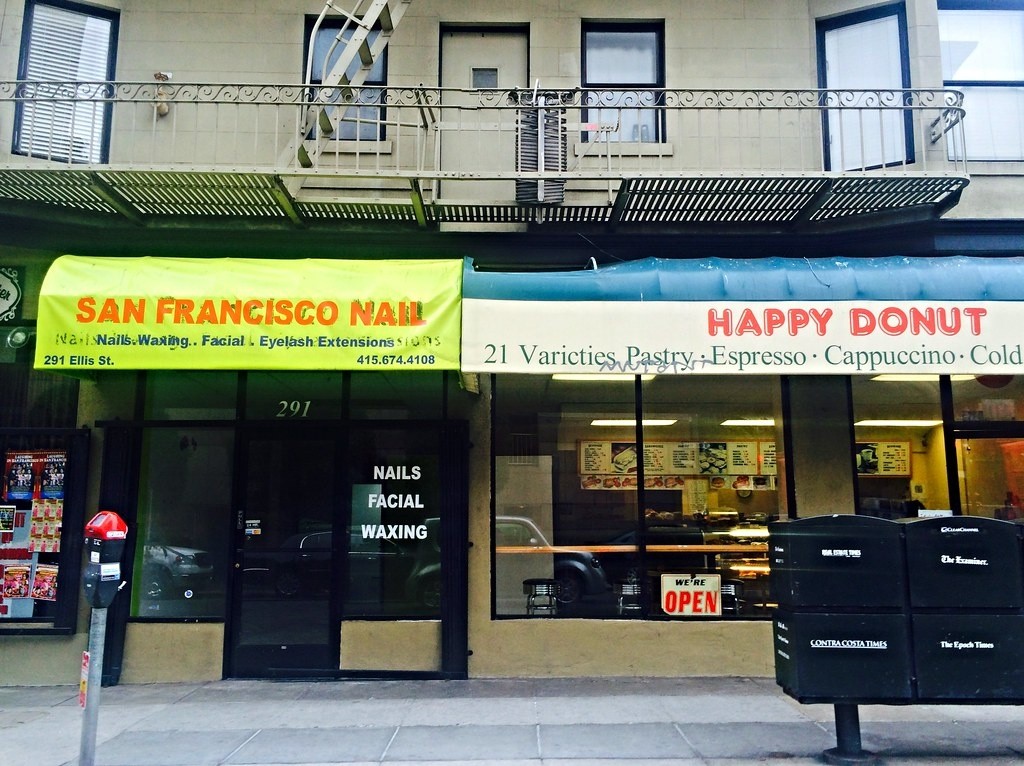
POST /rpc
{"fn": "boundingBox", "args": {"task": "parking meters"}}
[78,510,129,766]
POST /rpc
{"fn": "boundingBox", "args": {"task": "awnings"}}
[26,253,463,372]
[455,255,1024,379]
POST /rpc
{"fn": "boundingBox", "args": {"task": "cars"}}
[543,512,742,604]
[406,516,607,611]
[142,544,215,602]
[234,531,415,600]
